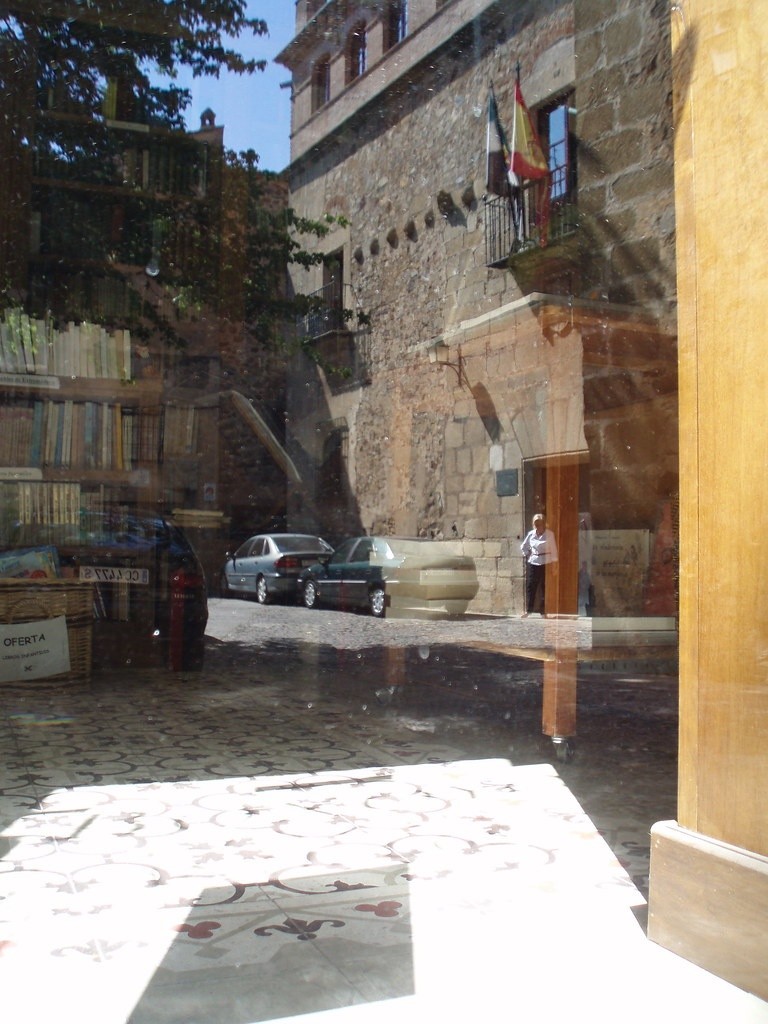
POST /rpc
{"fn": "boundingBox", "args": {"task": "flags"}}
[512,80,552,247]
[487,98,523,240]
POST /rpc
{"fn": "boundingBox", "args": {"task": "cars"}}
[297,536,479,618]
[15,507,209,672]
[219,533,335,605]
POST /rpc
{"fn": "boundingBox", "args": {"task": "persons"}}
[521,514,555,616]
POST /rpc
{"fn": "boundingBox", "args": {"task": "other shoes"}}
[520,612,531,618]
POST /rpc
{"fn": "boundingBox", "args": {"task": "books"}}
[0,55,220,622]
